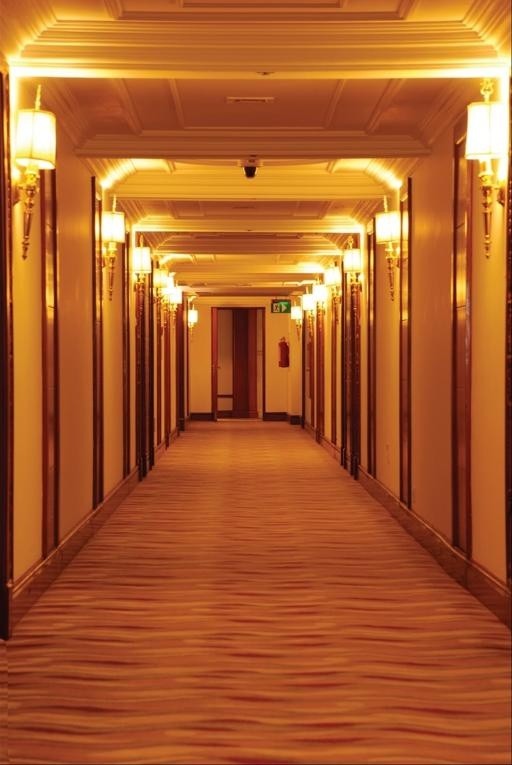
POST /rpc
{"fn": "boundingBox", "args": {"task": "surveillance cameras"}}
[237,159,263,178]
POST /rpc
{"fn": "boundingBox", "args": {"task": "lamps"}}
[134,233,197,337]
[464,76,509,256]
[373,195,401,302]
[11,83,58,260]
[291,236,363,342]
[100,192,125,302]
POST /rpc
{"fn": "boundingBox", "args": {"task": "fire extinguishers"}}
[278,337,290,367]
